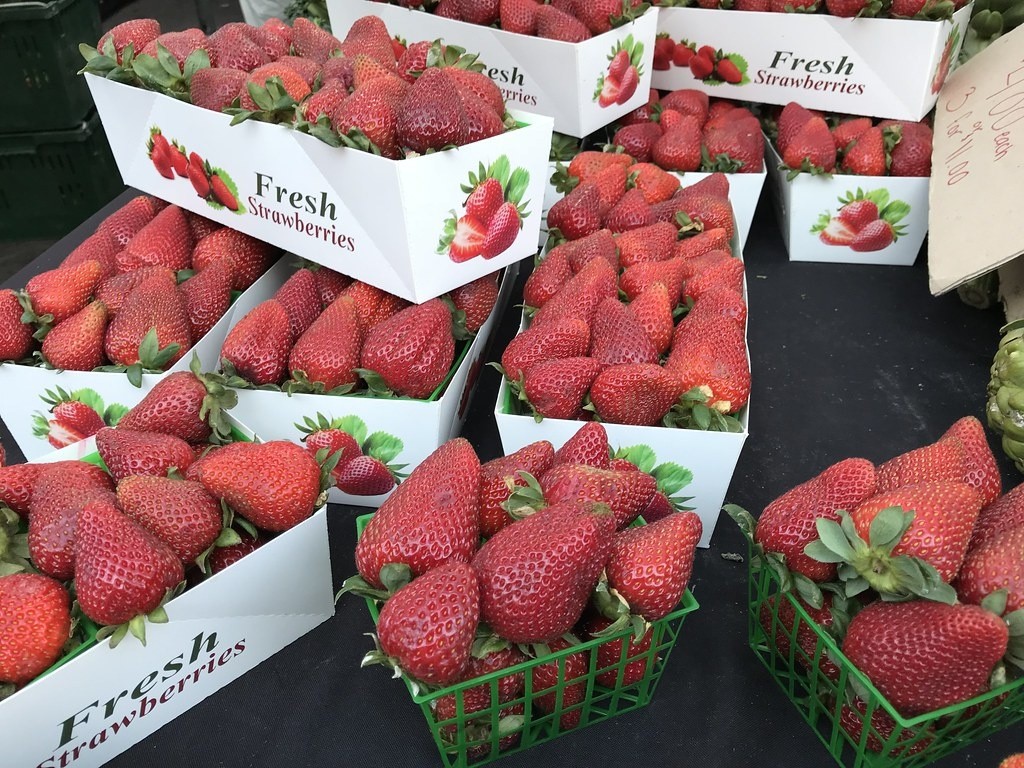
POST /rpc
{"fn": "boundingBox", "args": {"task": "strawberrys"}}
[0,1,1024,766]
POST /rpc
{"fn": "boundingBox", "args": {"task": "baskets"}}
[353,513,701,766]
[739,526,1024,768]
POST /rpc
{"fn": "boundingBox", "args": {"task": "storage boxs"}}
[0,407,337,768]
[648,0,975,123]
[0,186,289,461]
[492,198,755,551]
[325,0,661,140]
[541,158,768,256]
[73,60,553,304]
[210,260,510,512]
[758,125,930,265]
